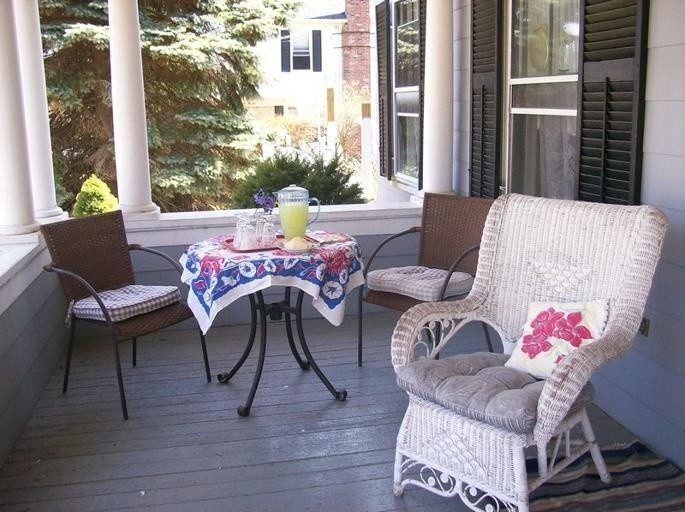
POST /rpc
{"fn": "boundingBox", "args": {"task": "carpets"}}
[485,438,684,511]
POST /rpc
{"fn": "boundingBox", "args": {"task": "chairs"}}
[38,209,211,421]
[358,193,495,367]
[390,194,668,512]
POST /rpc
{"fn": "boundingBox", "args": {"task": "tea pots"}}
[271,183,321,240]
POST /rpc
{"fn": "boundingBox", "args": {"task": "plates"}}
[273,238,320,254]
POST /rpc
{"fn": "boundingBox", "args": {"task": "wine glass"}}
[232,211,276,251]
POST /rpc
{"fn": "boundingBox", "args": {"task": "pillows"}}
[502,299,609,381]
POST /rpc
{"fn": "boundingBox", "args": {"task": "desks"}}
[177,230,366,416]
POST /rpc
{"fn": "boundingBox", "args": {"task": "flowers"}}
[253,188,276,214]
